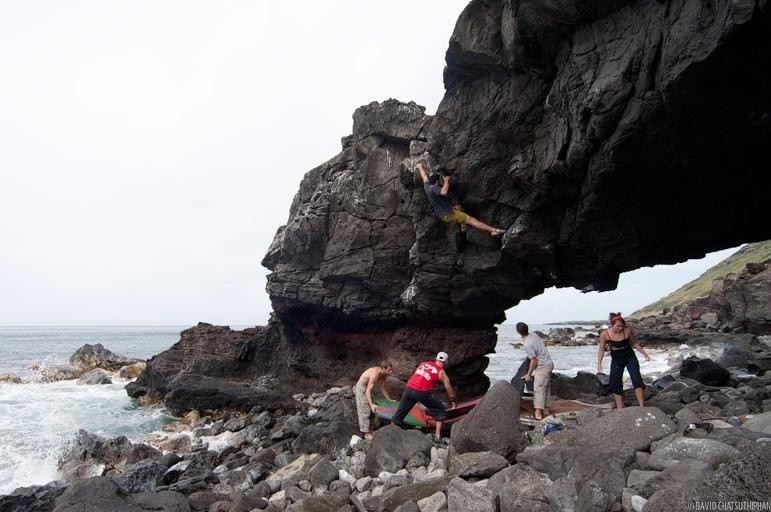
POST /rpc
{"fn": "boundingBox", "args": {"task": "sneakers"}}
[434,435,448,444]
[461,226,471,233]
[491,228,508,237]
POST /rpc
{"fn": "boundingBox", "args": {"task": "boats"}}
[372,330,551,430]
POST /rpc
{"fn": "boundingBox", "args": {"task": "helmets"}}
[437,351,449,364]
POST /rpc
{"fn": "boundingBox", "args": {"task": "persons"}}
[392,351,458,447]
[415,163,508,238]
[516,321,555,422]
[596,312,651,409]
[355,360,397,440]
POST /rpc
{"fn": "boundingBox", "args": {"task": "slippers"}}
[525,414,543,420]
